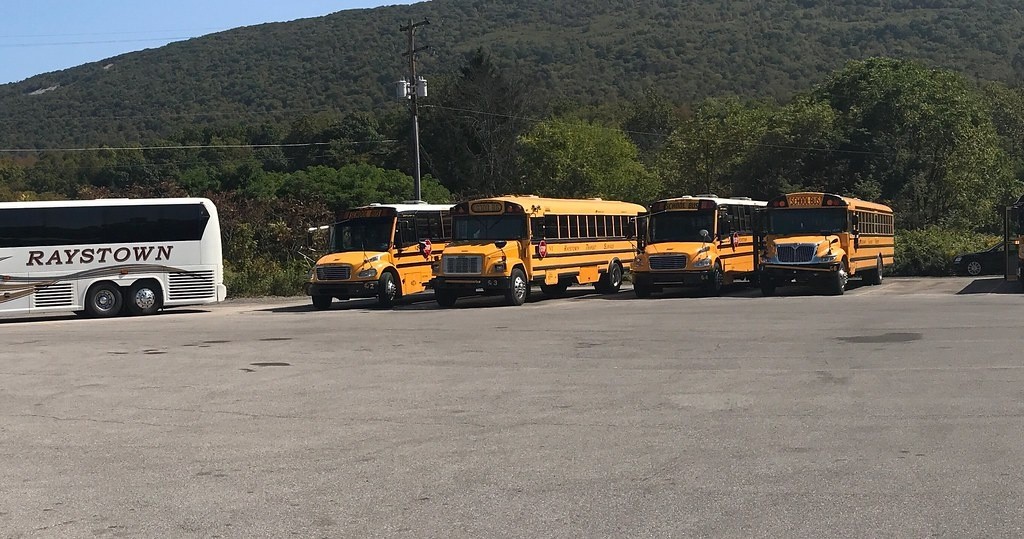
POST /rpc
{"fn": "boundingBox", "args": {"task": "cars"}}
[953,241,1016,276]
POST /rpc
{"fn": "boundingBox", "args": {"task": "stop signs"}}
[538,240,546,256]
[732,233,739,248]
[423,240,432,255]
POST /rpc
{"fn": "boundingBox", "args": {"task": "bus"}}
[417,193,649,308]
[624,195,775,297]
[1003,195,1024,285]
[752,192,895,295]
[298,201,480,310]
[0,198,227,322]
